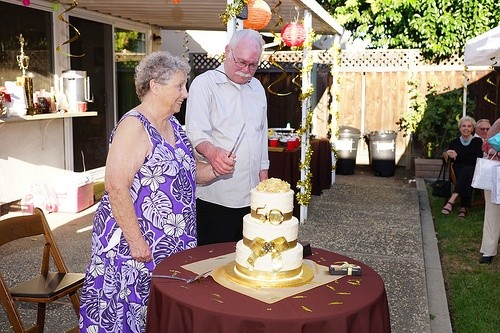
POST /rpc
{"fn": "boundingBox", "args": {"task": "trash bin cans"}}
[335,125,361,175]
[366,130,397,177]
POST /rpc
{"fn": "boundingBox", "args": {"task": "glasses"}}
[231,47,259,69]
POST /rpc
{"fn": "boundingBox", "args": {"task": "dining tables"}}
[144,242,391,333]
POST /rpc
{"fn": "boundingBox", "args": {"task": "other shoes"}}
[480,255,495,264]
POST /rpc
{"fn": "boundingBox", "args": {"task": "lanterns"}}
[281,23,306,47]
[242,0,272,31]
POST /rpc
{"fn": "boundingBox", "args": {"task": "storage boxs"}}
[21,174,94,213]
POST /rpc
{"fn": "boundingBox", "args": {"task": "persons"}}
[186,30,269,250]
[441,117,500,265]
[77,54,234,333]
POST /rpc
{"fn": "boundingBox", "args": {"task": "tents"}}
[463,28,500,119]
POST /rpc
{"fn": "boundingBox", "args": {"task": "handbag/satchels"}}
[432,179,451,198]
[491,152,500,204]
[470,148,500,191]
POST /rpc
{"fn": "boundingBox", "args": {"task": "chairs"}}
[442,151,486,216]
[0,208,87,333]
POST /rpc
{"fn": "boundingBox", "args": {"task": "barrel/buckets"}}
[59,69,86,114]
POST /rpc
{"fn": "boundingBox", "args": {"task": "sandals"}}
[457,210,468,219]
[441,201,454,214]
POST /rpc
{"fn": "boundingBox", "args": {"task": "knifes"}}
[227,123,247,158]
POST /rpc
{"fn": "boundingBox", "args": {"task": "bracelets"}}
[212,166,219,178]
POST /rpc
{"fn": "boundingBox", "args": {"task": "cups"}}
[269,138,299,151]
[79,102,88,112]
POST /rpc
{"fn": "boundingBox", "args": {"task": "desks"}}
[263,139,331,197]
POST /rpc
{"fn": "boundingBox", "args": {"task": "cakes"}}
[234,178,303,281]
